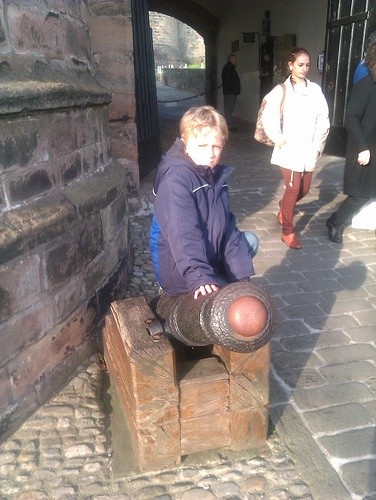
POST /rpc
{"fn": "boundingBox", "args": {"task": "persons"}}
[150,106,258,300]
[326,32,376,243]
[221,54,241,132]
[261,47,330,249]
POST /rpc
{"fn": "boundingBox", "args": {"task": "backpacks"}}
[252,82,286,147]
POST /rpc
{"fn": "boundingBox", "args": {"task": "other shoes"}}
[277,199,285,226]
[280,232,302,250]
[325,213,345,244]
